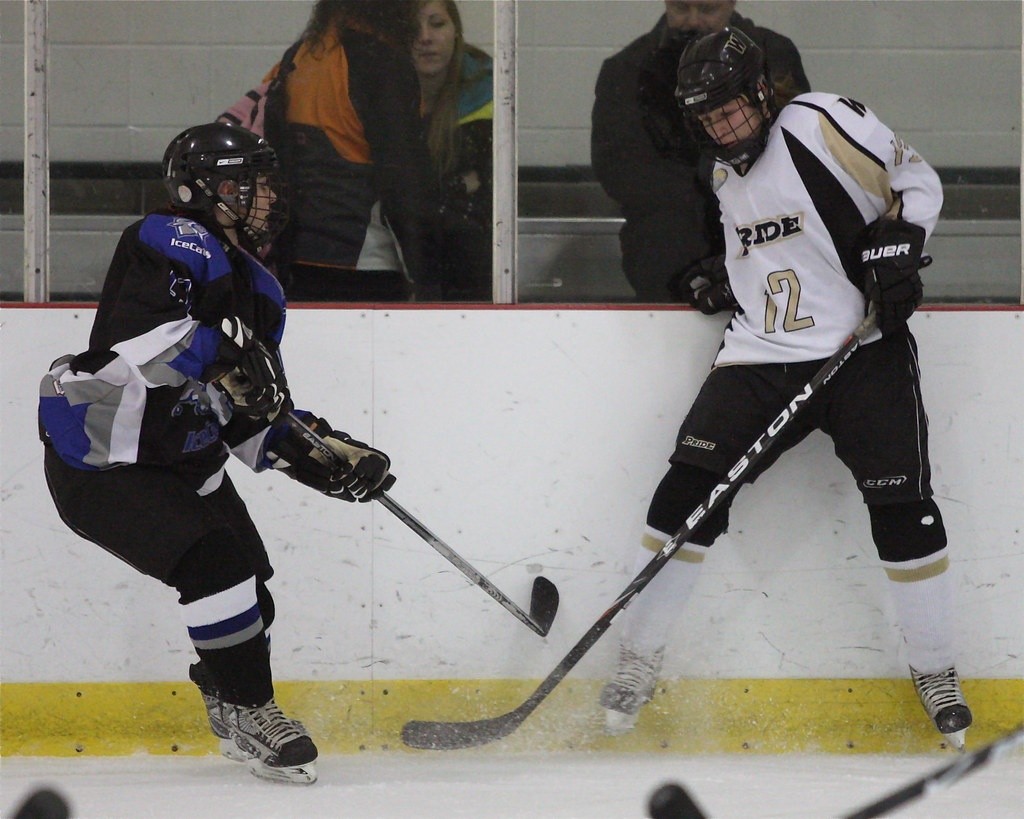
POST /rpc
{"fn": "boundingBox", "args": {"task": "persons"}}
[38,122,396,785]
[600,29,972,753]
[220,0,495,301]
[590,0,812,302]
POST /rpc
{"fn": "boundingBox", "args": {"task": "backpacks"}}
[213,40,306,263]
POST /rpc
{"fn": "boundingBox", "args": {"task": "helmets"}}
[163,122,277,210]
[673,26,767,113]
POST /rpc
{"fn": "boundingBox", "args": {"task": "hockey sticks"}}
[283,407,560,638]
[648,718,1024,819]
[401,257,932,752]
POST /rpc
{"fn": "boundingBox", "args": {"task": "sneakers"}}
[598,641,665,734]
[912,666,973,751]
[203,690,317,784]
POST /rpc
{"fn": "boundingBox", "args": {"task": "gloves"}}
[266,418,390,503]
[681,262,735,315]
[864,222,924,328]
[200,334,290,415]
